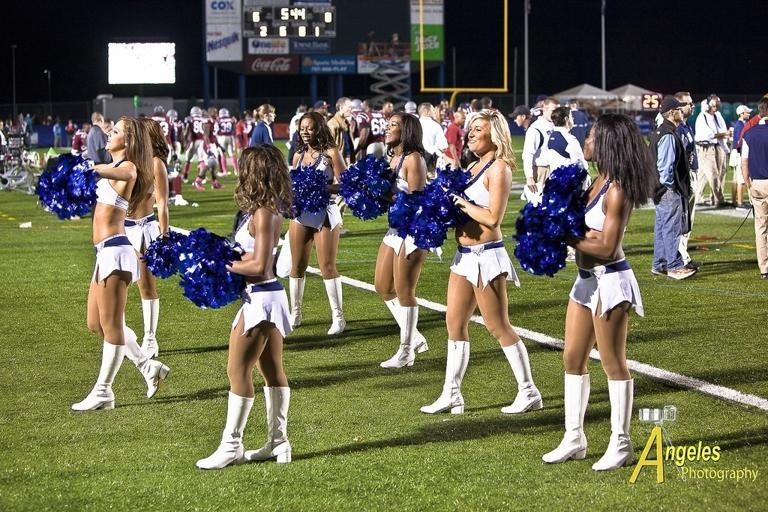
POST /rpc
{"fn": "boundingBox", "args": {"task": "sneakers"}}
[695,203,710,208]
[651,267,667,276]
[174,195,189,206]
[339,229,349,235]
[715,202,735,209]
[760,274,768,279]
[192,181,206,191]
[736,203,753,211]
[667,267,696,279]
[216,171,223,177]
[211,180,225,190]
[181,174,189,183]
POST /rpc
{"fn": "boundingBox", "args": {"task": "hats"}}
[508,105,530,118]
[239,109,249,119]
[659,97,687,114]
[536,95,546,101]
[736,105,753,116]
[314,100,330,108]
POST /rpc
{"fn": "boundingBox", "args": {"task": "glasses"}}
[675,108,681,111]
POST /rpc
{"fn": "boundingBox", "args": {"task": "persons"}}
[729,105,753,209]
[418,109,544,414]
[213,108,240,178]
[192,107,228,191]
[542,114,660,471]
[549,108,591,263]
[122,119,167,366]
[52,119,64,149]
[534,95,547,117]
[234,109,252,175]
[356,98,384,158]
[440,108,466,168]
[324,99,353,164]
[374,113,428,368]
[458,97,492,117]
[250,103,276,146]
[563,98,591,150]
[521,98,561,204]
[289,104,308,142]
[741,97,768,279]
[182,106,203,183]
[151,105,175,164]
[87,110,115,165]
[71,115,168,410]
[0,121,9,175]
[416,102,448,158]
[652,98,698,279]
[673,92,697,269]
[166,109,184,171]
[195,146,293,468]
[737,94,768,156]
[380,103,398,116]
[288,111,348,334]
[157,161,192,207]
[508,104,537,140]
[71,122,92,157]
[694,95,730,206]
[65,120,77,147]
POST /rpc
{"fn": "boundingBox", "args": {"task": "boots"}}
[71,341,126,411]
[290,276,306,329]
[322,275,346,335]
[125,325,138,356]
[380,306,418,369]
[196,392,255,470]
[420,340,470,414]
[591,380,634,471]
[384,297,428,354]
[542,372,590,462]
[500,339,543,414]
[140,298,160,359]
[124,324,170,398]
[244,386,292,464]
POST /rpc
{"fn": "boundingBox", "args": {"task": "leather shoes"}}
[685,261,700,272]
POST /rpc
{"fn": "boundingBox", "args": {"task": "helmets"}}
[190,106,202,117]
[350,99,362,112]
[405,101,416,114]
[167,110,177,120]
[219,108,229,117]
[154,106,164,113]
